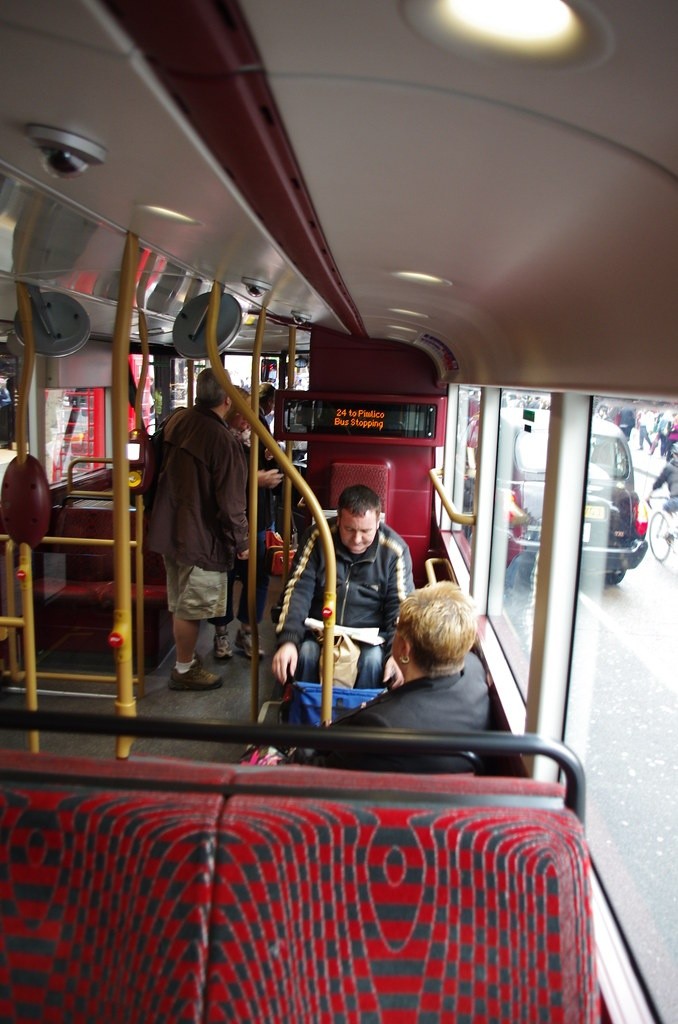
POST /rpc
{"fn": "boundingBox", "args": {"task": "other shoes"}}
[664,530,675,541]
[638,447,668,458]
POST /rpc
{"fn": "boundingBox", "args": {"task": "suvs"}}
[464,407,649,586]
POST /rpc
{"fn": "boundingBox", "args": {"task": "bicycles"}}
[644,495,678,562]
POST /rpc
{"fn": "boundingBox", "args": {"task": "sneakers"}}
[168,650,222,690]
[214,630,233,660]
[234,629,265,660]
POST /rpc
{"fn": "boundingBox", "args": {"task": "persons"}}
[272,482,417,698]
[143,368,311,691]
[309,582,488,777]
[467,391,678,552]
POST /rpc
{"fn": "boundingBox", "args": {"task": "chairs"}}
[309,748,482,776]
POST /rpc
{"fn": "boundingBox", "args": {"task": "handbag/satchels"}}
[614,412,622,426]
[277,681,388,726]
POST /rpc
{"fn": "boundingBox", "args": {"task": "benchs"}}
[30,494,173,612]
[0,703,602,1023]
